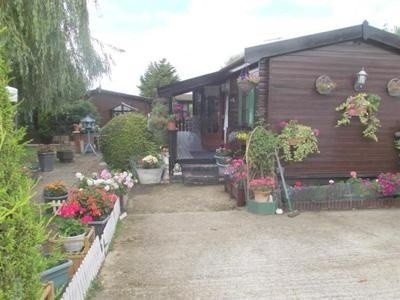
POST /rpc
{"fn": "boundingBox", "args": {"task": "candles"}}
[43,180,68,212]
[59,219,87,253]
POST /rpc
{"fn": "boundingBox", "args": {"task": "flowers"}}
[60,187,117,222]
[248,177,276,192]
[278,119,320,162]
[141,155,157,168]
[215,146,231,157]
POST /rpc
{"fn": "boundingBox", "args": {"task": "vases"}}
[290,137,304,146]
[216,156,230,164]
[254,191,270,202]
[136,167,164,184]
[89,215,109,235]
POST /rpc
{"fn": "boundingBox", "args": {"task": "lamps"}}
[354,66,368,91]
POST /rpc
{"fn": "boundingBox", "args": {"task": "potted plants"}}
[40,245,73,291]
[335,93,380,143]
[168,115,177,130]
[37,145,56,171]
[56,143,75,161]
